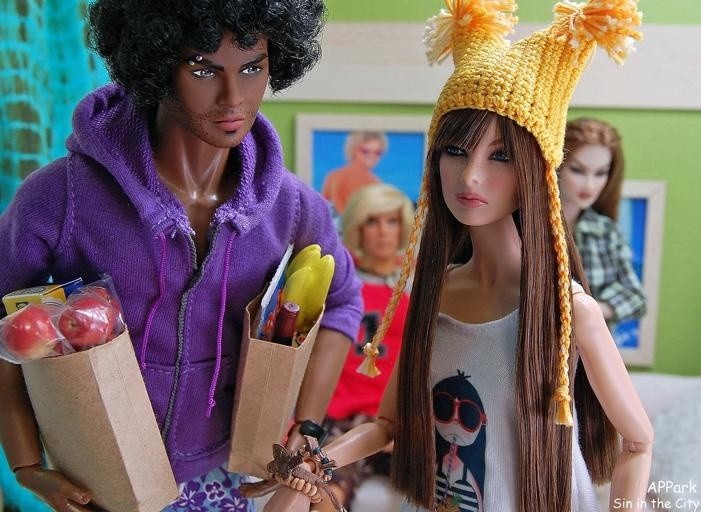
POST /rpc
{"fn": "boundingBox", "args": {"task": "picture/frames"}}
[610,180,664,367]
[295,112,434,227]
